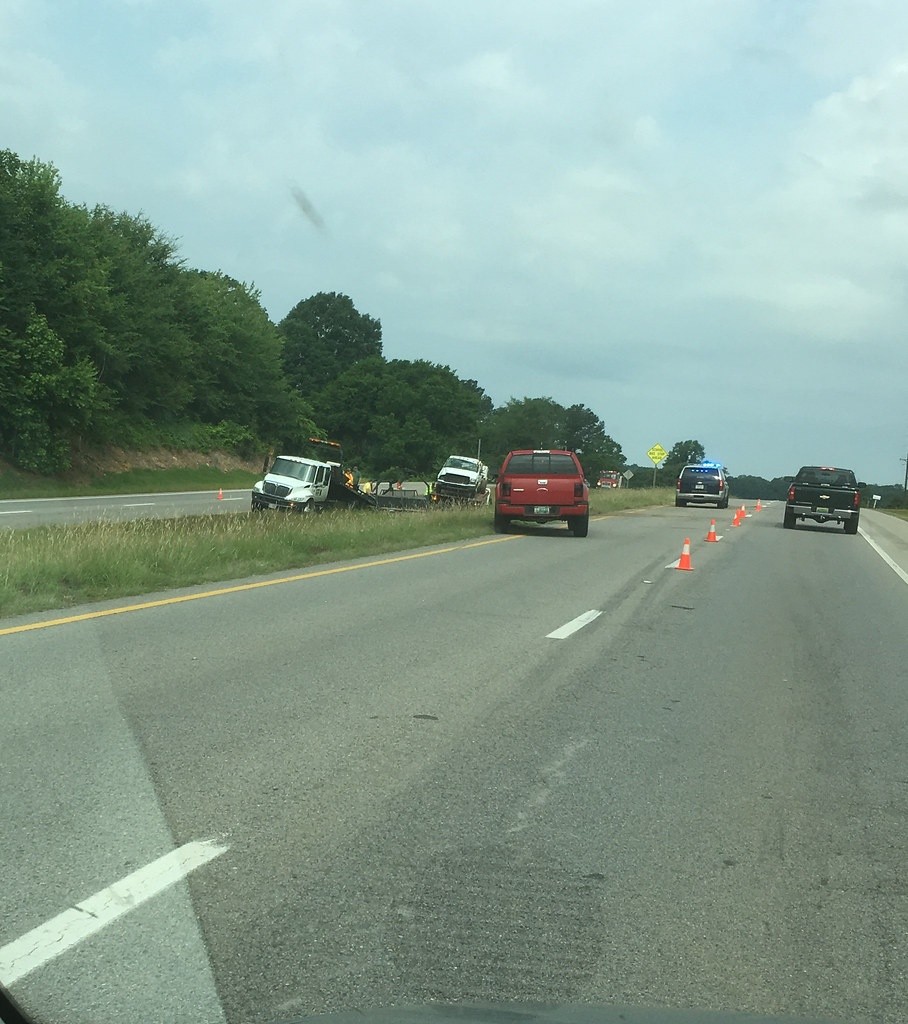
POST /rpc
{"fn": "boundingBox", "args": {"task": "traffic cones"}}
[217,488,224,500]
[755,499,762,511]
[674,538,695,570]
[706,518,719,542]
[730,511,741,527]
[739,504,748,518]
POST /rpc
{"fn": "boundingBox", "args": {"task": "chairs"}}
[451,462,462,467]
[836,475,847,484]
[805,472,815,481]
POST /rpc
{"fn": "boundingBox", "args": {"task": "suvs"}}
[494,445,590,537]
[675,461,730,509]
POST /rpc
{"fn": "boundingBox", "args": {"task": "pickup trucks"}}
[784,466,866,534]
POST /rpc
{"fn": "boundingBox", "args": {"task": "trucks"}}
[436,455,489,499]
[250,438,379,513]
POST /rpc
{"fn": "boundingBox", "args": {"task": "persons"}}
[424,481,435,500]
[342,465,402,495]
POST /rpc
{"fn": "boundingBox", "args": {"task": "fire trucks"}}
[596,470,626,488]
